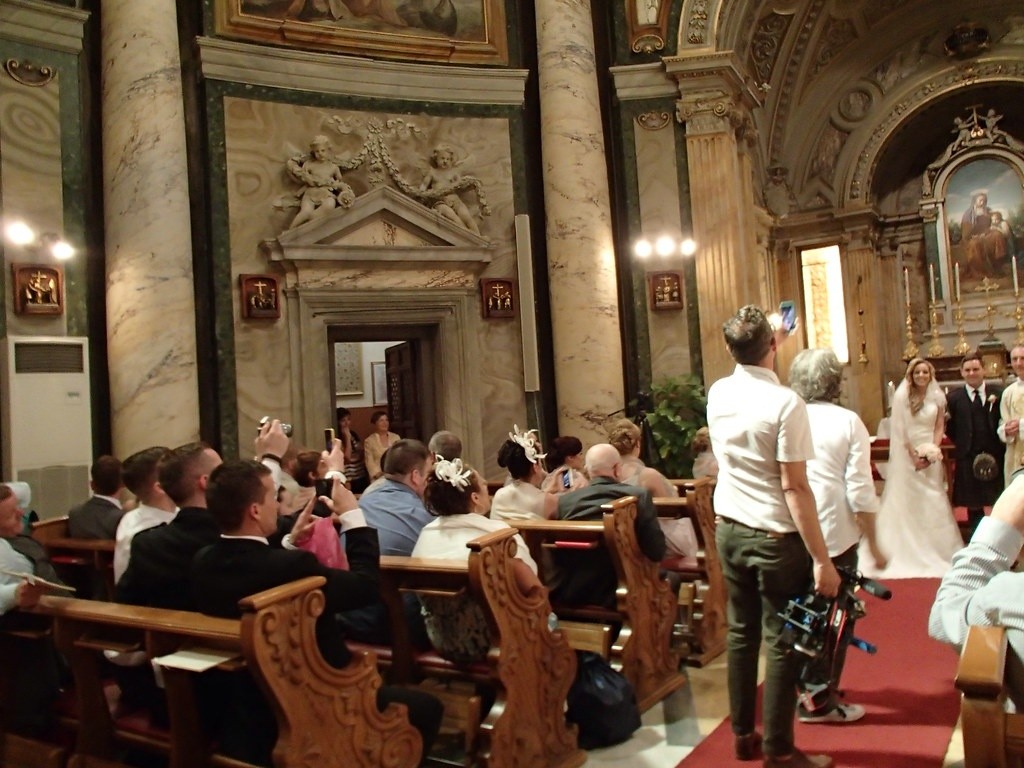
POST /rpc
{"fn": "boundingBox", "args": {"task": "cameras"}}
[256,417,293,438]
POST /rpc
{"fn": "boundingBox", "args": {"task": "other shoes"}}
[736,730,767,758]
[761,743,835,768]
[797,698,867,724]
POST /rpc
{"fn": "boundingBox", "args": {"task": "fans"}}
[988,394,996,411]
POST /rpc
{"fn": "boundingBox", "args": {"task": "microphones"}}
[837,567,893,600]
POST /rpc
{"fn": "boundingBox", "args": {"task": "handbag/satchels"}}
[297,519,346,569]
[568,649,642,753]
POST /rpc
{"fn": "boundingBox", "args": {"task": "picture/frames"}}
[213,1,509,67]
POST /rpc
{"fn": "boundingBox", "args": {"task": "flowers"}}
[913,443,944,473]
[432,454,473,494]
[508,424,548,464]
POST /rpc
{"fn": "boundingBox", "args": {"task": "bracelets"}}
[815,558,832,567]
[262,452,282,464]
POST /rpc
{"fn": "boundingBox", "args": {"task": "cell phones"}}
[563,469,570,490]
[315,478,333,499]
[780,299,795,333]
[325,429,335,455]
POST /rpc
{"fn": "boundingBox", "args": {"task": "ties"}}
[973,389,982,410]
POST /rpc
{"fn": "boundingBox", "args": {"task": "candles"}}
[905,267,911,307]
[1012,256,1019,295]
[930,264,935,303]
[955,262,961,303]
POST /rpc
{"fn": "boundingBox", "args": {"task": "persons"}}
[855,358,965,579]
[929,473,1024,715]
[281,134,343,234]
[997,344,1024,490]
[421,142,480,237]
[68,442,448,768]
[358,430,537,577]
[337,407,369,494]
[364,412,401,484]
[489,417,698,567]
[707,308,888,768]
[0,480,75,619]
[944,353,1003,541]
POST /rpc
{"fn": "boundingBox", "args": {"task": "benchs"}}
[955,623,1024,768]
[0,479,729,768]
